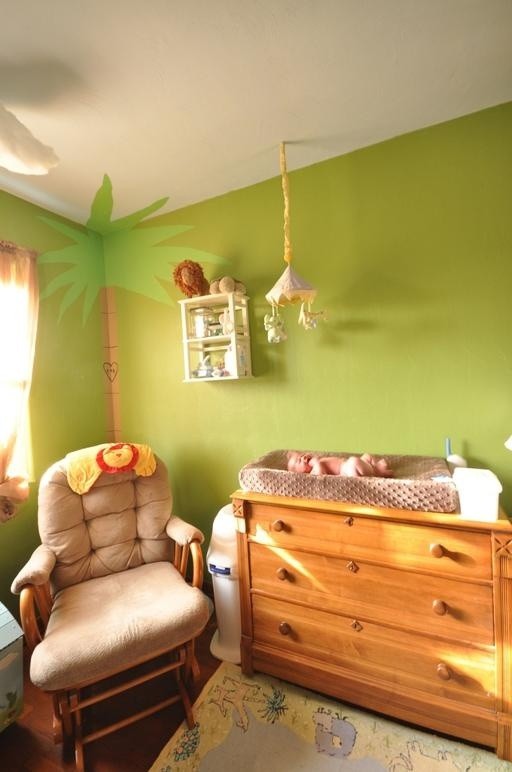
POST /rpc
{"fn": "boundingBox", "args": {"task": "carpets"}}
[150,661,511,772]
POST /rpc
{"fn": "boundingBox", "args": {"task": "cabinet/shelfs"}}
[176,291,255,383]
[228,489,511,763]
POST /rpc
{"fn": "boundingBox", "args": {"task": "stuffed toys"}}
[209,271,247,295]
[173,260,210,298]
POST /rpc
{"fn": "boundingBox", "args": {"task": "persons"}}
[283,451,395,478]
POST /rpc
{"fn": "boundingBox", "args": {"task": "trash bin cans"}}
[206,503,245,665]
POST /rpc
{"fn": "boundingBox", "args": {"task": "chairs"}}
[10,444,214,771]
